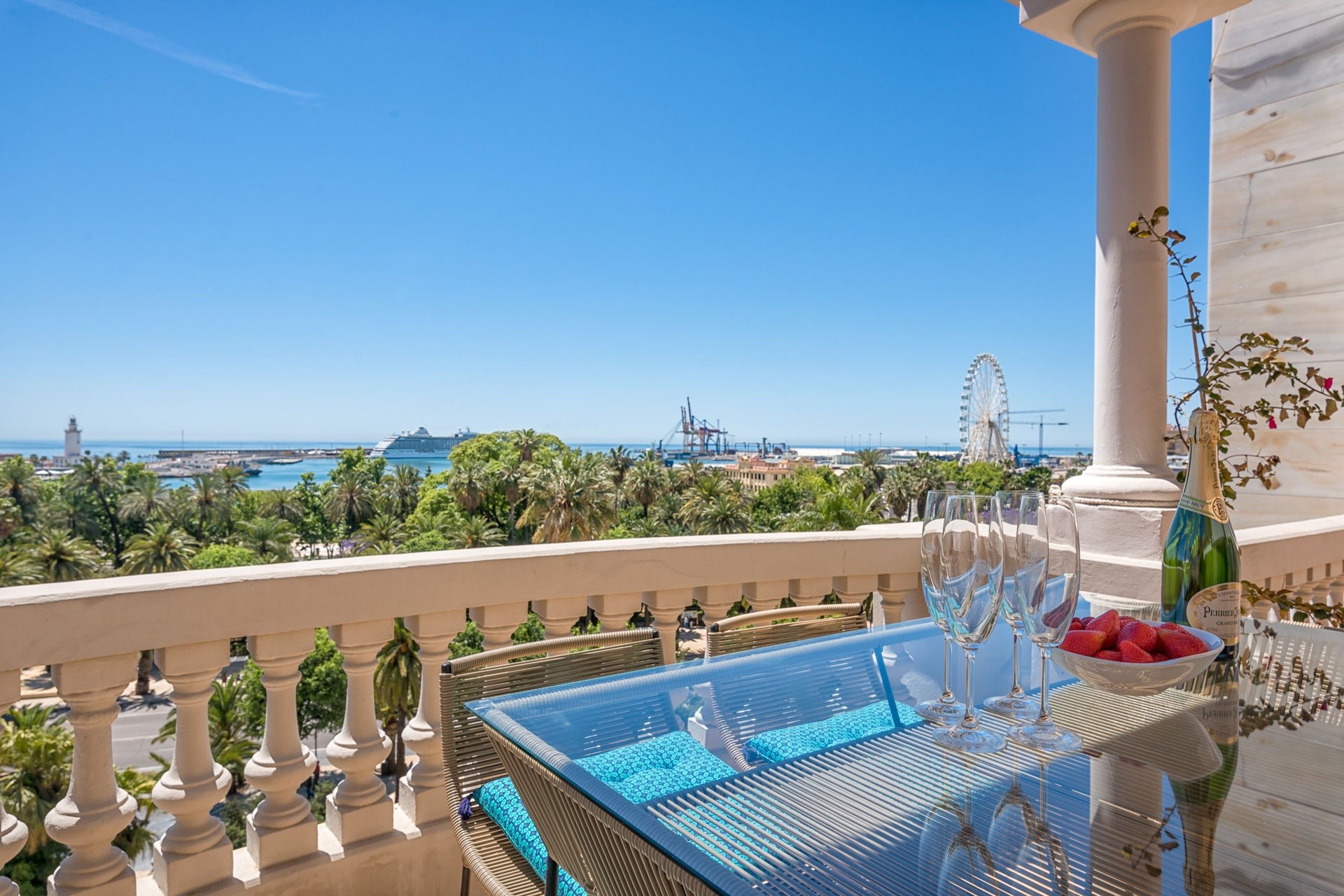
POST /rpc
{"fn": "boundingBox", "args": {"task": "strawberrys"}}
[1055,609,1209,663]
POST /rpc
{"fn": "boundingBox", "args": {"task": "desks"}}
[463,581,1344,896]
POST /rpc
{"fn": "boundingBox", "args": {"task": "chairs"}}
[706,593,929,770]
[438,628,801,896]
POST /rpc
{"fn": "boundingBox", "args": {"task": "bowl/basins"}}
[1036,683,1226,777]
[1050,619,1224,696]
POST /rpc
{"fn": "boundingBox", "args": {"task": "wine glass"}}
[933,496,1007,754]
[913,488,983,725]
[980,494,1053,721]
[916,728,1082,895]
[1007,494,1083,753]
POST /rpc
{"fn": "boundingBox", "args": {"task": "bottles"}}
[1161,411,1241,661]
[1169,659,1242,895]
